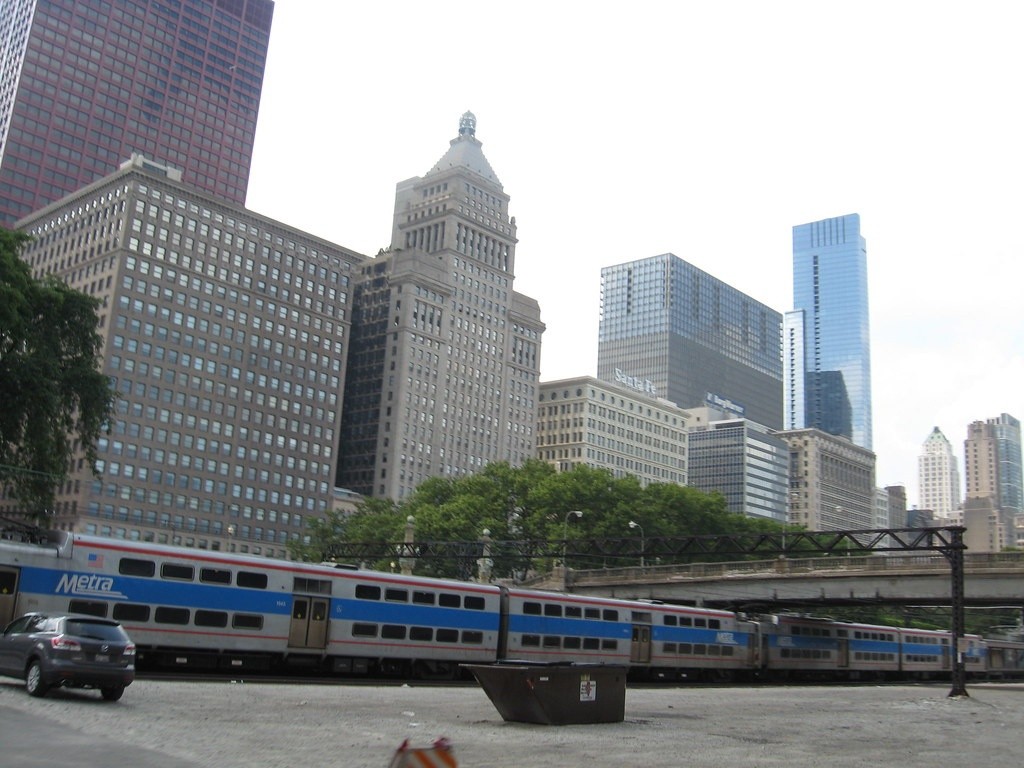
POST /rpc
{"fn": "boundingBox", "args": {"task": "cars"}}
[0,612,137,701]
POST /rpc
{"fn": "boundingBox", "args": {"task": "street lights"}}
[629,521,645,566]
[563,510,584,566]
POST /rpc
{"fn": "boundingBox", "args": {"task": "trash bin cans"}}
[458,658,629,726]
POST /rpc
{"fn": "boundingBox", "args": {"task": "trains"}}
[0,533,1024,682]
